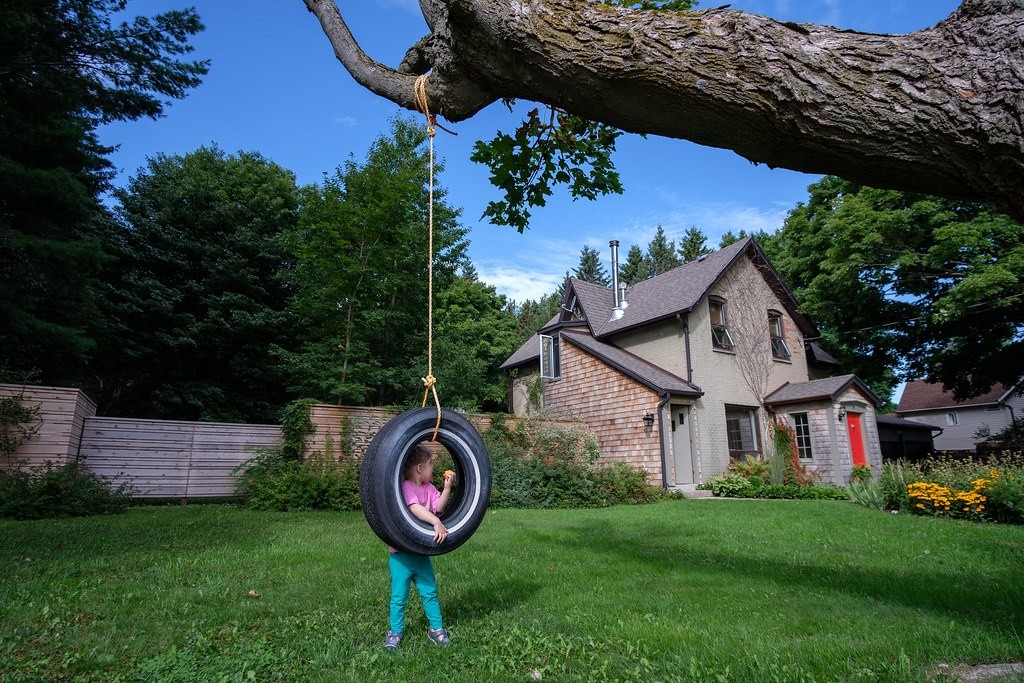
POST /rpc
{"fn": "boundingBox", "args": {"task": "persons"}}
[386,446,454,648]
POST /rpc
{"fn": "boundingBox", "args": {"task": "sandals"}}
[427,629,449,646]
[385,631,403,649]
